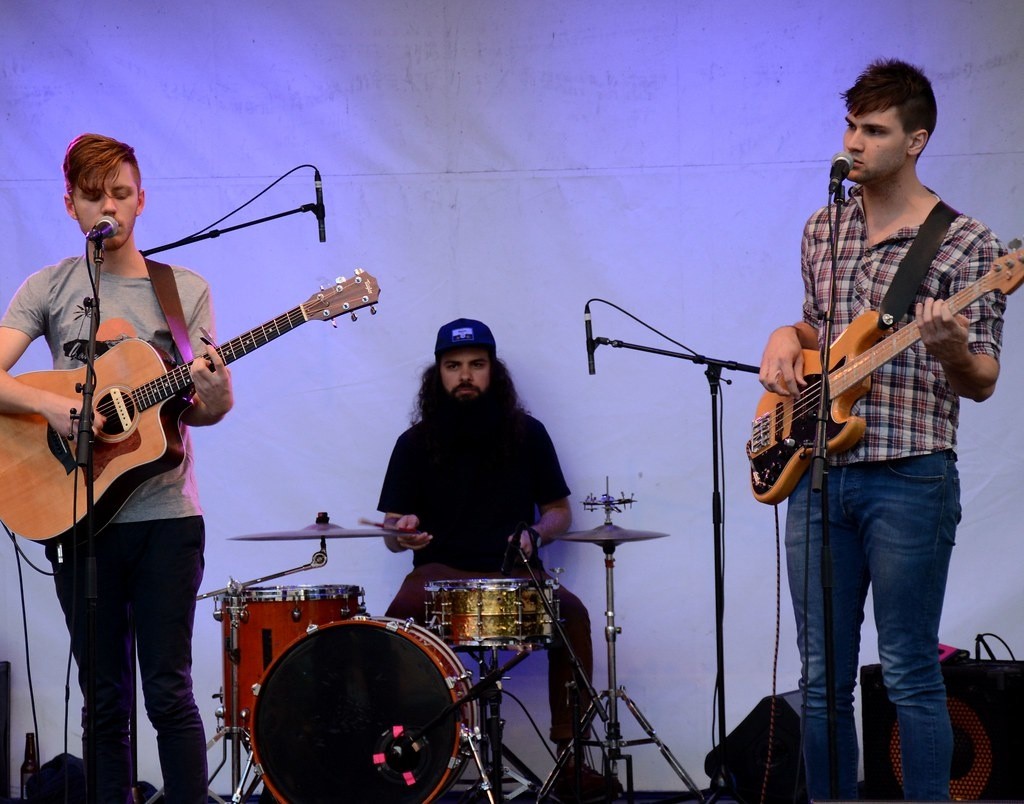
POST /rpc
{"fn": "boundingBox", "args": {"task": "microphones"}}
[86,216,118,241]
[828,151,854,195]
[314,170,326,242]
[391,738,409,759]
[584,305,596,374]
[501,523,523,578]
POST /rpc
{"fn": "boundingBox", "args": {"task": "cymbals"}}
[225,527,417,542]
[549,527,670,545]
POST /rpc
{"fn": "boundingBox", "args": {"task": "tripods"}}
[597,336,766,804]
[535,542,706,804]
[148,539,329,804]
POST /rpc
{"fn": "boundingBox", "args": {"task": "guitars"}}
[750,238,1024,505]
[0,269,382,549]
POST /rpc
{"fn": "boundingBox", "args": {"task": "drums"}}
[245,618,474,804]
[218,584,363,743]
[423,578,561,652]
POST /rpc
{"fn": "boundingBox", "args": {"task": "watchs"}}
[527,526,544,557]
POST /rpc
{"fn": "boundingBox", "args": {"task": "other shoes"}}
[553,759,624,800]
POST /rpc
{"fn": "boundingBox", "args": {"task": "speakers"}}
[704,688,811,804]
[26,753,87,804]
[860,659,1024,801]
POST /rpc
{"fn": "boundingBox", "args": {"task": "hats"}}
[434,319,496,357]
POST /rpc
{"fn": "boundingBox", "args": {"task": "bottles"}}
[19,732,39,804]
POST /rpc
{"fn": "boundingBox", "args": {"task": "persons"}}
[758,56,1008,804]
[375,318,622,804]
[0,131,234,804]
